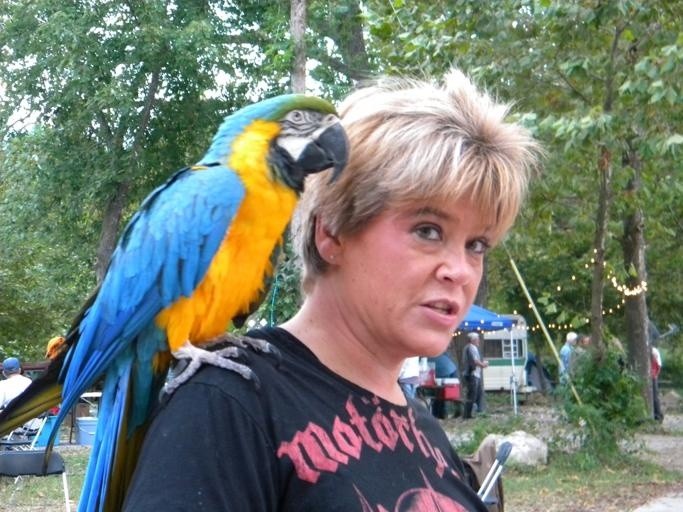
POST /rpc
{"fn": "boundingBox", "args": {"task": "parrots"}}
[1,91,352,512]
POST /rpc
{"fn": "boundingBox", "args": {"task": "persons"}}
[651,346,664,420]
[0,357,43,436]
[121,68,550,512]
[462,332,489,419]
[559,332,591,387]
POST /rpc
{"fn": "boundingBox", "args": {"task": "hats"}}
[2,357,20,372]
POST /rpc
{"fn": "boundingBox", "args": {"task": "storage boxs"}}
[435,377,461,402]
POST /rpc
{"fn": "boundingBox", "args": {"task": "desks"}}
[68,392,101,446]
